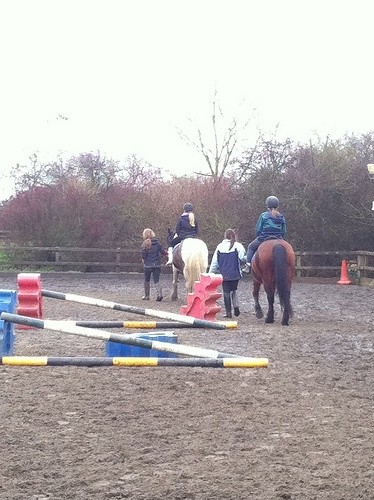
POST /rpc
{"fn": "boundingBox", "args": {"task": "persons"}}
[141,228,169,302]
[242,196,287,273]
[166,203,196,266]
[208,228,247,318]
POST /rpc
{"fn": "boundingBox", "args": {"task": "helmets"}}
[183,203,193,212]
[265,196,279,209]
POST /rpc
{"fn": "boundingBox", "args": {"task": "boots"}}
[232,290,240,315]
[165,247,173,266]
[223,291,232,317]
[142,282,150,300]
[155,282,163,301]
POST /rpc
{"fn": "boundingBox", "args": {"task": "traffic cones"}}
[337,259,353,284]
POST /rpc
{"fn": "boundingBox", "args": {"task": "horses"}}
[165,228,208,301]
[251,240,295,326]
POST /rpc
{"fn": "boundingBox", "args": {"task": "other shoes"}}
[242,264,251,273]
